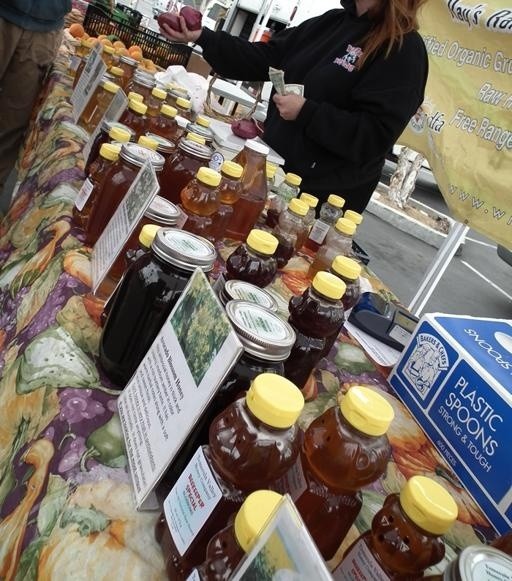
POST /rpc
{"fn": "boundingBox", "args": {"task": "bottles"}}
[58,43,512,581]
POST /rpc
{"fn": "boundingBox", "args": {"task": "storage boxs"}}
[186,49,212,79]
[386,313,512,540]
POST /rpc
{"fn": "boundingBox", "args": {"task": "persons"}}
[0,0,74,193]
[154,1,429,217]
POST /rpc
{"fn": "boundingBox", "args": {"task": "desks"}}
[203,72,267,117]
[1,34,512,579]
